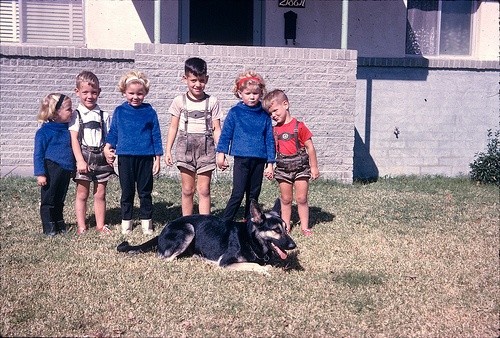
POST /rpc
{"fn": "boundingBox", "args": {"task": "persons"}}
[104,72,163,236]
[165,57,229,217]
[68,71,116,235]
[34,93,77,234]
[262,89,320,236]
[216,71,276,222]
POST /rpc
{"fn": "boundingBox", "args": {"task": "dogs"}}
[116,198,296,270]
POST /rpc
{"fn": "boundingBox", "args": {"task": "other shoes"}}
[96,226,112,234]
[140,219,154,235]
[122,219,133,235]
[78,228,87,235]
[301,228,312,236]
